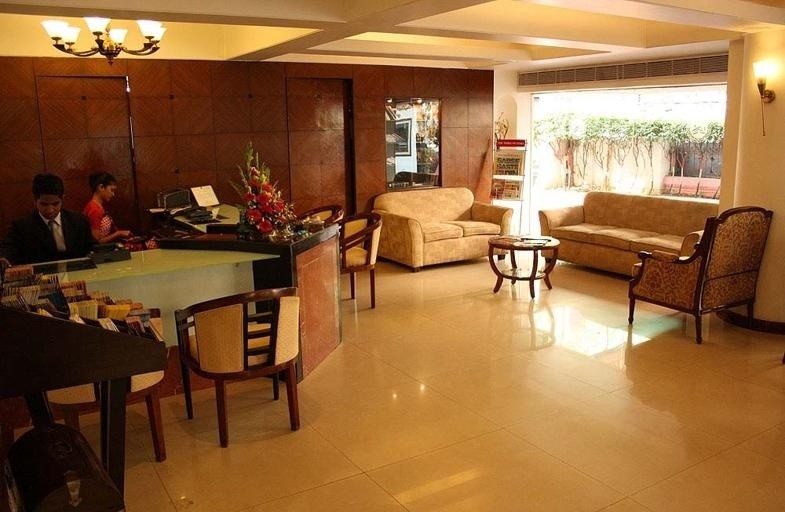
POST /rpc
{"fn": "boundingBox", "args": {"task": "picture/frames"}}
[395,118,412,157]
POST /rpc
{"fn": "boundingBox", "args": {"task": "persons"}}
[0,172,97,270]
[83,172,134,247]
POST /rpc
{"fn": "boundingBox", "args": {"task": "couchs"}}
[538,192,720,278]
[371,187,514,272]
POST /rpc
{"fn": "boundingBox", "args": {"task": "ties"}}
[49,220,67,251]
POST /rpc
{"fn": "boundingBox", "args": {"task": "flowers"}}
[229,140,310,234]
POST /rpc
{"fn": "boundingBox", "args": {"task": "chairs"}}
[174,287,300,448]
[297,204,344,228]
[628,207,774,344]
[324,212,383,308]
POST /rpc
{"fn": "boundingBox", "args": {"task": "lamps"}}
[753,61,776,136]
[40,16,167,66]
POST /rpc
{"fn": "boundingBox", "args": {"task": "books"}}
[521,233,552,246]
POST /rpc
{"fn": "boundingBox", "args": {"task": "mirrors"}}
[386,98,442,190]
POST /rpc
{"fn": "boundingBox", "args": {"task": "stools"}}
[46,370,166,462]
